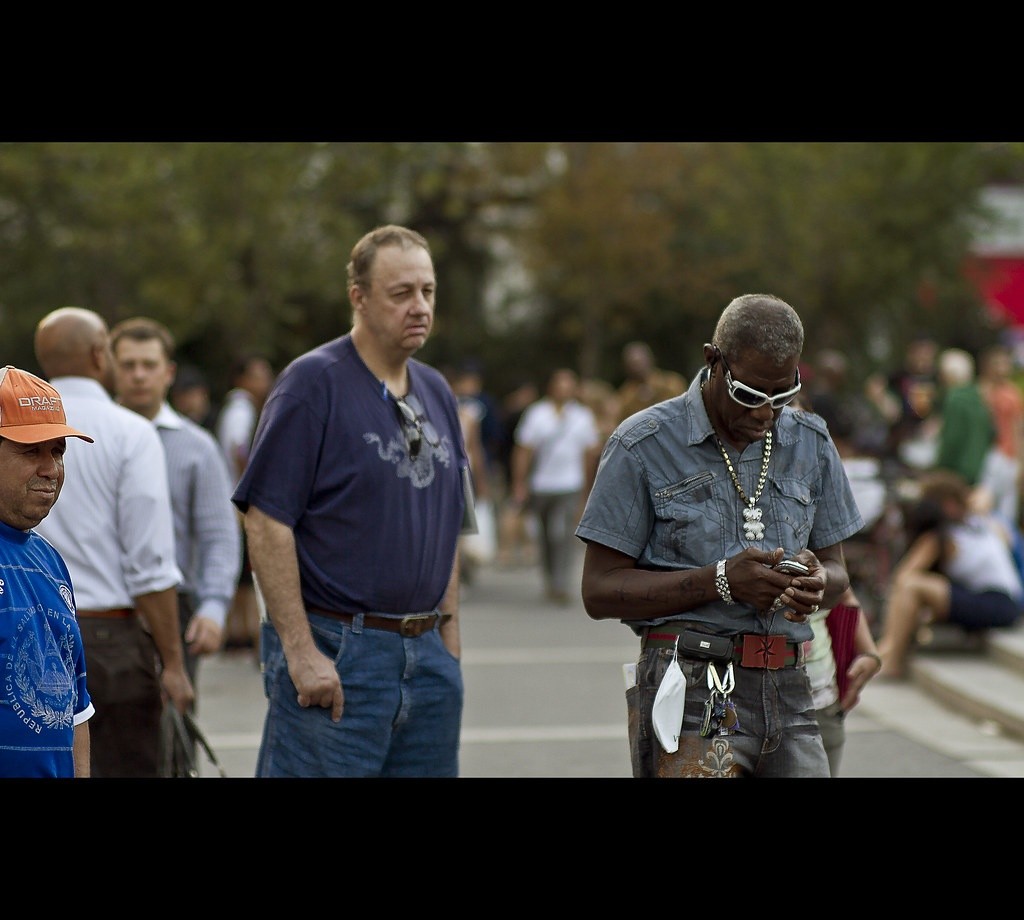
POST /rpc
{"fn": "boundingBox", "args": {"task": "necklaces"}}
[699,379,773,542]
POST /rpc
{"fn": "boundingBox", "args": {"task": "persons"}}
[26,306,273,778]
[0,362,96,778]
[450,336,687,607]
[785,328,1024,778]
[229,223,480,778]
[575,292,866,778]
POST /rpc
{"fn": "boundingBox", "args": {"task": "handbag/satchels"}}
[826,606,860,699]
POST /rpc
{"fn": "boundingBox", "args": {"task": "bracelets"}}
[715,557,737,608]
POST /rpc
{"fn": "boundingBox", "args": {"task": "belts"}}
[77,607,139,619]
[645,625,804,670]
[302,600,452,638]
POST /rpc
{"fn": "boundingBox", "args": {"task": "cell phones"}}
[770,560,810,579]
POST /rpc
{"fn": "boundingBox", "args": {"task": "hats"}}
[0,364,96,445]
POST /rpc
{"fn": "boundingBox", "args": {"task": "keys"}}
[699,685,740,738]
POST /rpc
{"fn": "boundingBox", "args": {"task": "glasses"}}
[715,346,801,410]
